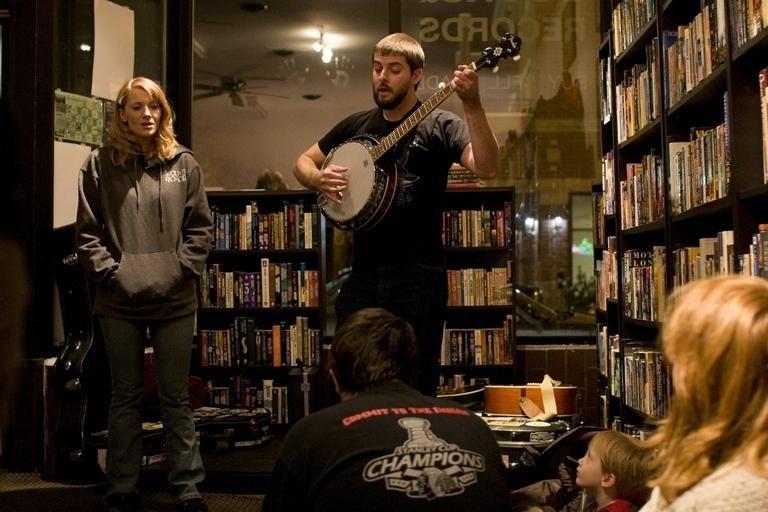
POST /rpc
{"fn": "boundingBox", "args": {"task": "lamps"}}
[313,23,333,64]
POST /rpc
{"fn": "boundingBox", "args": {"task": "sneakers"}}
[106,491,140,512]
[176,497,208,512]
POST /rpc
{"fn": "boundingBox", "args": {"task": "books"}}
[596,1,768,444]
[193,202,318,423]
[442,207,515,389]
[445,163,481,189]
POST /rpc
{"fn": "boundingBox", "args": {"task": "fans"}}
[191,30,291,108]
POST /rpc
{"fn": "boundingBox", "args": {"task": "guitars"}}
[483,381,579,417]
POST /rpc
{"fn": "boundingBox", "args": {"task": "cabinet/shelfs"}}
[327,186,516,416]
[594,0,768,439]
[192,186,326,434]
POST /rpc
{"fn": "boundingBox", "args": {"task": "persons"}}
[74,76,215,512]
[292,30,502,410]
[636,274,768,511]
[576,430,649,511]
[258,306,513,511]
[257,169,287,191]
